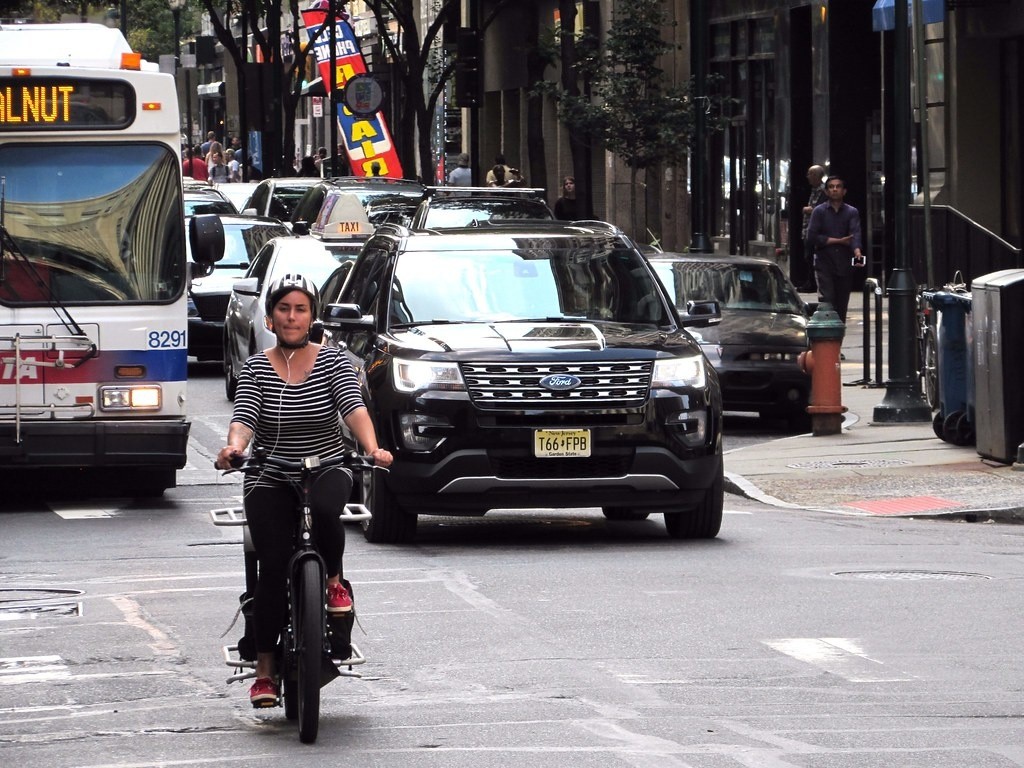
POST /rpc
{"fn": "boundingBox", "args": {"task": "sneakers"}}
[325,582,352,612]
[248,676,277,704]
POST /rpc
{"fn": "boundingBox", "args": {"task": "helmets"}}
[266,273,320,321]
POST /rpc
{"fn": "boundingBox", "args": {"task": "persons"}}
[807,175,862,359]
[796,166,829,292]
[217,273,393,704]
[554,176,590,221]
[181,130,260,185]
[449,153,527,189]
[292,144,351,178]
[364,162,386,183]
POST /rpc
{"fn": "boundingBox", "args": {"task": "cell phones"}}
[852,256,866,266]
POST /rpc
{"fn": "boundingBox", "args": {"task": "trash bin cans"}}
[924,290,974,443]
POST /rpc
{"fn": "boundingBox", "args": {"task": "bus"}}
[0,17,225,497]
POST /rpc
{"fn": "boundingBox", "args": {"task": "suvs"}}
[319,218,724,543]
[411,187,554,230]
[291,175,429,245]
[182,180,244,359]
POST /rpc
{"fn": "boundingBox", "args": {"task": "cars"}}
[184,174,258,210]
[642,250,812,429]
[224,230,366,399]
[239,177,324,223]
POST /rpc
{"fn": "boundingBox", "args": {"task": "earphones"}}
[267,323,273,330]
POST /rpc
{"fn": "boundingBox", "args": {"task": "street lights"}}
[168,0,186,73]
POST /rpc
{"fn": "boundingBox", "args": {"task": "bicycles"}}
[915,285,940,411]
[215,446,390,744]
